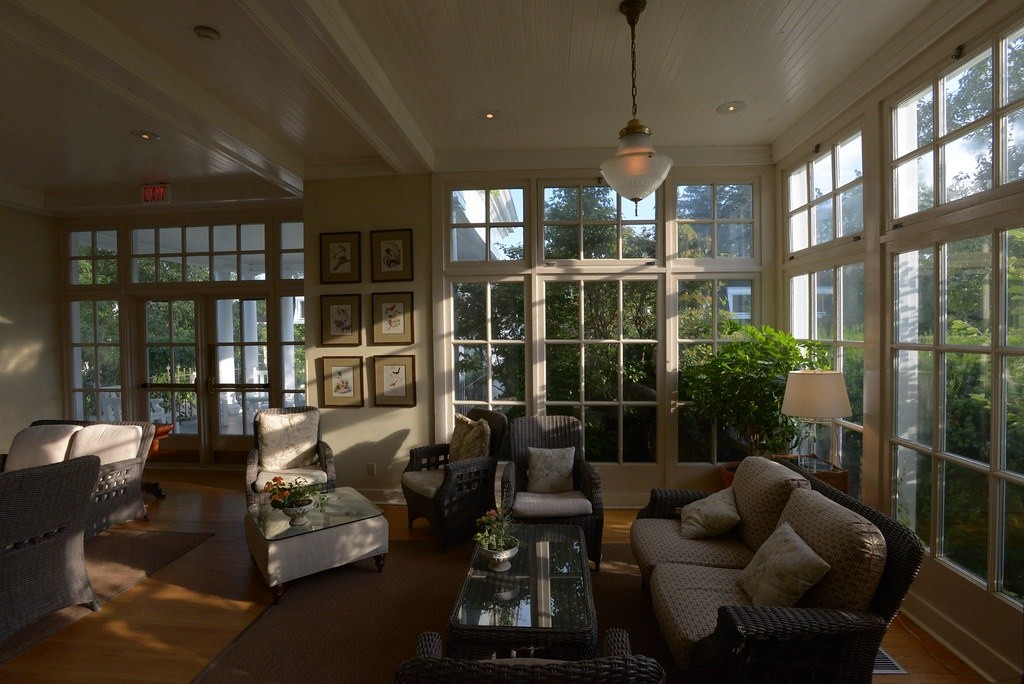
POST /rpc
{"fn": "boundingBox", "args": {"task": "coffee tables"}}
[447,523,599,660]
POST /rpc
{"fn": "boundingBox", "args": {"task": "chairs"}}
[253,366,267,398]
[502,415,604,572]
[394,628,667,684]
[245,406,337,511]
[94,385,166,423]
[0,455,103,645]
[401,408,508,554]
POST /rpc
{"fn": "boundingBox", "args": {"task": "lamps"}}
[596,0,673,217]
[782,370,852,465]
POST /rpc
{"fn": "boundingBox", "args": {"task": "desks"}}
[141,422,173,499]
[238,392,268,424]
[245,487,389,600]
[769,452,849,494]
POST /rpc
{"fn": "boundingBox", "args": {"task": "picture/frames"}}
[374,355,416,408]
[372,291,414,346]
[318,231,362,284]
[322,356,364,407]
[320,294,362,346]
[369,228,414,282]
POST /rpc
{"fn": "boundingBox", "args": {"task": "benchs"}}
[0,419,155,541]
[630,455,925,684]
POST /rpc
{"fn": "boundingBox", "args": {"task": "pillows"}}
[448,413,490,480]
[258,411,320,473]
[526,446,575,494]
[736,522,831,608]
[680,486,741,540]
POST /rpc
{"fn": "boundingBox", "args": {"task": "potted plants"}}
[680,325,831,488]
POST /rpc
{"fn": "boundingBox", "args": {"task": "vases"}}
[282,498,314,525]
[478,536,519,572]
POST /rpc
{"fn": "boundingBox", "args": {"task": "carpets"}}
[0,528,216,665]
[189,539,688,684]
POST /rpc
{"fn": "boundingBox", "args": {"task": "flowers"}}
[472,504,520,551]
[259,477,329,512]
